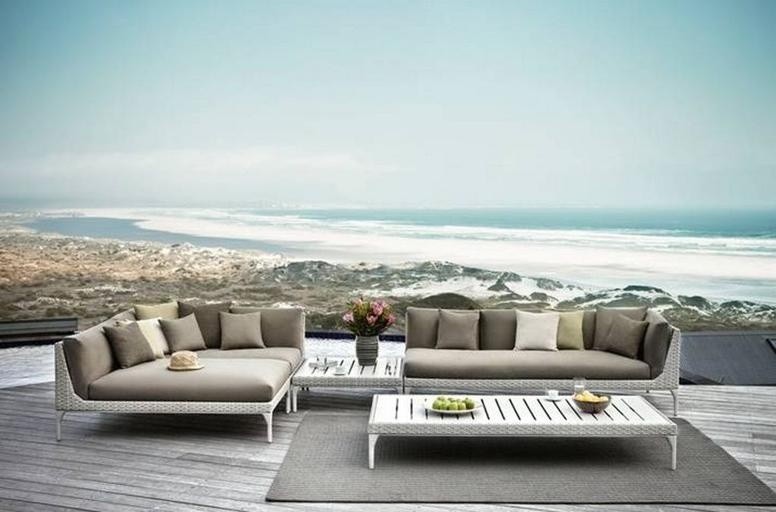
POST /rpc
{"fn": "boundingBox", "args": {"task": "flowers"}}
[341,295,395,337]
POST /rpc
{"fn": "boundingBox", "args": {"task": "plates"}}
[425,402,482,416]
[545,397,564,402]
[332,372,346,375]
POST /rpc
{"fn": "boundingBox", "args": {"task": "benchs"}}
[402,312,681,416]
[53,312,306,444]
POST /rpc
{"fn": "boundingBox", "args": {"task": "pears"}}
[575,390,608,402]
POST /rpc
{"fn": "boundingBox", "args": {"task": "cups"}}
[547,389,557,398]
[334,367,344,373]
[316,350,327,370]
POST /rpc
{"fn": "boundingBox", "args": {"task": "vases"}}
[355,336,379,366]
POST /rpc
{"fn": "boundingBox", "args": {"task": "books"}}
[309,360,337,369]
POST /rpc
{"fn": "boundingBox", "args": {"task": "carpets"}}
[264,412,776,504]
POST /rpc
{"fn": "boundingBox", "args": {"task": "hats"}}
[167,348,204,373]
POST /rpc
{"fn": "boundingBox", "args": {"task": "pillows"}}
[406,306,672,379]
[61,300,305,401]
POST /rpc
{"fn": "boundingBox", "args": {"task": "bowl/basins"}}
[573,395,612,415]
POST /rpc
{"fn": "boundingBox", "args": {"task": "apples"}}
[432,396,474,410]
